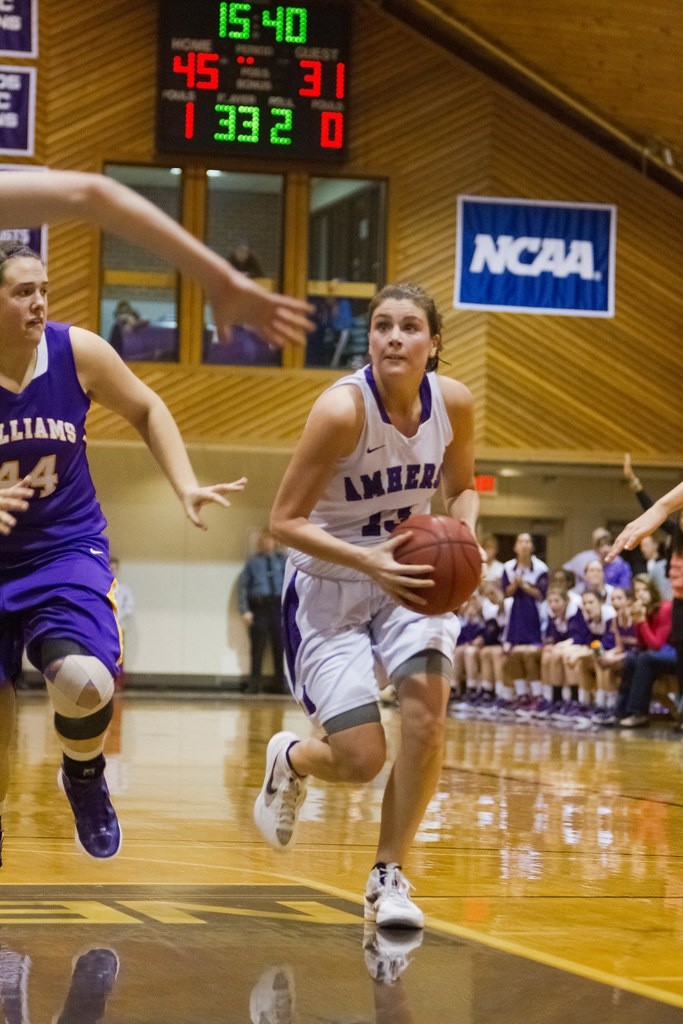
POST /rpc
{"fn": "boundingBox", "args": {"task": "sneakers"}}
[364,862,424,929]
[49,943,121,1024]
[253,732,309,857]
[447,685,649,729]
[250,965,296,1024]
[363,920,424,982]
[58,756,122,860]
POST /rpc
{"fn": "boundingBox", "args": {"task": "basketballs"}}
[387,514,483,616]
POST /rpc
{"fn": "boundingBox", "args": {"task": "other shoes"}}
[273,680,291,694]
[244,684,259,694]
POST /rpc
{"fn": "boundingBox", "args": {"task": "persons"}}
[238,526,289,696]
[107,556,134,623]
[374,452,683,732]
[0,165,314,351]
[249,919,425,1024]
[0,941,120,1024]
[431,711,682,907]
[254,281,489,929]
[106,245,354,370]
[0,238,251,860]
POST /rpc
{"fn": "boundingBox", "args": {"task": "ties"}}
[266,556,276,596]
